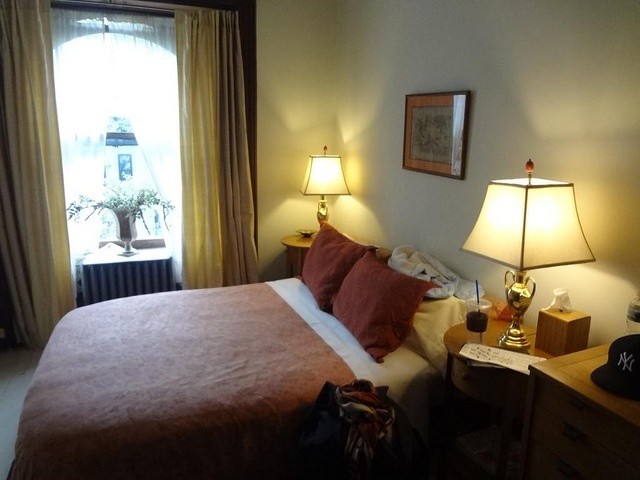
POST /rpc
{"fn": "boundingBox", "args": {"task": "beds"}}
[6,219,478,479]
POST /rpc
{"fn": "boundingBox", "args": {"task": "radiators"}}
[80,257,176,305]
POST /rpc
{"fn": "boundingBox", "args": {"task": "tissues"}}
[535,289,591,357]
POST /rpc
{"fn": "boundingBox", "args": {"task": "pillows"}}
[331,249,444,363]
[301,221,380,314]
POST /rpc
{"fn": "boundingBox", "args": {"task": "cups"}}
[466,299,492,347]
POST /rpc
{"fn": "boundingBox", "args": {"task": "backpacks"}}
[291,380,429,480]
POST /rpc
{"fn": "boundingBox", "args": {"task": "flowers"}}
[66,181,175,237]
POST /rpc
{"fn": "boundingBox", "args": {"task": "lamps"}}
[460,155,596,354]
[299,143,351,228]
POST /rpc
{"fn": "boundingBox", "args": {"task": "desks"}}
[441,318,555,479]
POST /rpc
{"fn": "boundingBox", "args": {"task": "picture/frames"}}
[402,89,471,181]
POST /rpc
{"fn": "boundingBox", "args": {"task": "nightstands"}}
[281,233,317,279]
[514,347,640,480]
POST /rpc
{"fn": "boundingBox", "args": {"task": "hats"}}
[591,334,640,400]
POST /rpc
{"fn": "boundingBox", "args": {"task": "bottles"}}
[624,291,639,336]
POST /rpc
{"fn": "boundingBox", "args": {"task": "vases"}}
[116,209,138,257]
